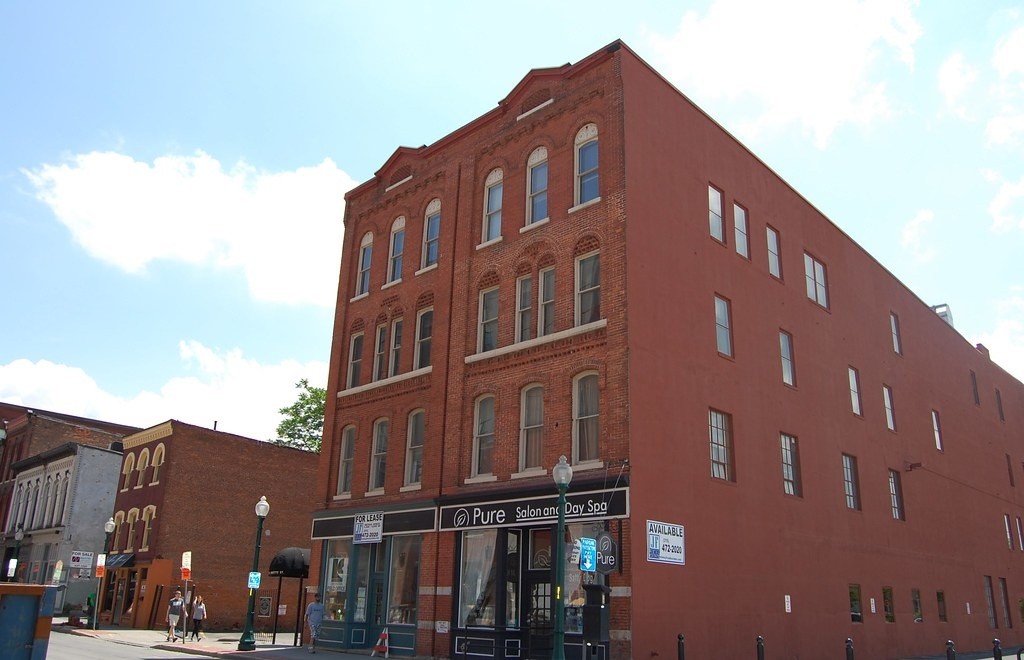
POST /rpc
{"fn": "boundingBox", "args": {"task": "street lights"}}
[86,516,116,629]
[235,495,268,651]
[9,528,24,583]
[551,454,574,658]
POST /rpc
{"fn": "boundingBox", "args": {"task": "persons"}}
[304,593,324,654]
[165,591,188,643]
[191,595,206,642]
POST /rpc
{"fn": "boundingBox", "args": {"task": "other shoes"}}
[173,638,177,642]
[308,648,316,654]
[191,637,194,641]
[197,638,201,641]
[167,637,170,641]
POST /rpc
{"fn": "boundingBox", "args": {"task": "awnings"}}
[105,554,136,567]
